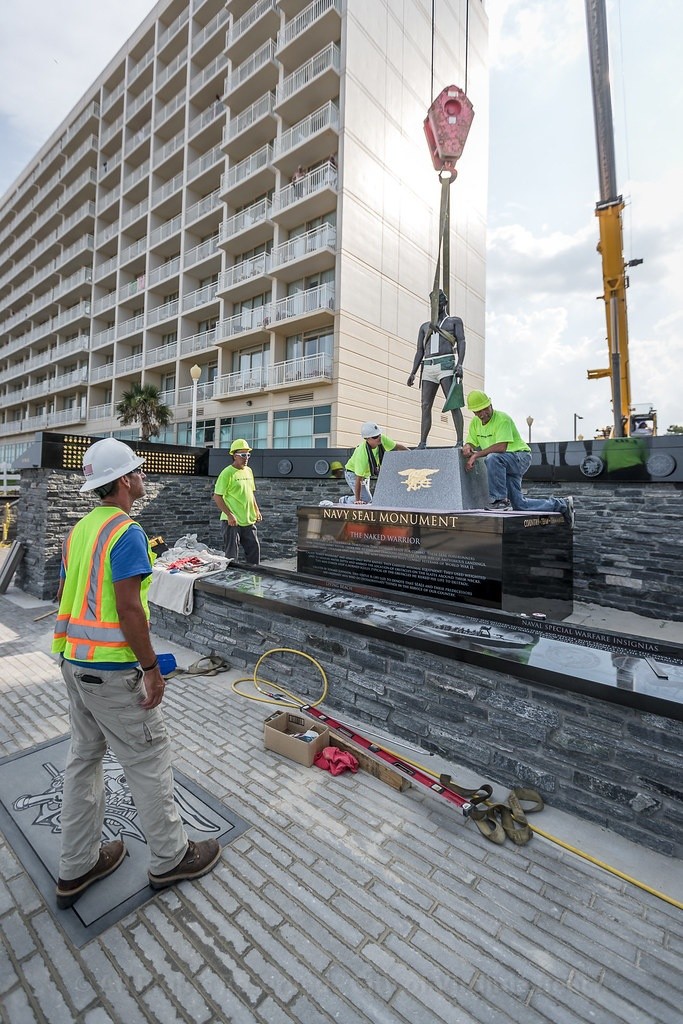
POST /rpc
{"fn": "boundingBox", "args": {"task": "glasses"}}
[127,467,141,474]
[236,452,250,458]
[371,434,380,439]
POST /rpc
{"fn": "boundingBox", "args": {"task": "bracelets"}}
[142,662,157,671]
[142,659,157,669]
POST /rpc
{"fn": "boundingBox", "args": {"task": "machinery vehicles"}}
[422,0,659,442]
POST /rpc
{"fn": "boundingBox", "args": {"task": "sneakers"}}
[484,499,513,511]
[148,838,221,889]
[56,840,126,896]
[560,496,575,530]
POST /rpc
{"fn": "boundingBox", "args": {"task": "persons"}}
[230,305,239,333]
[338,422,410,504]
[291,165,305,200]
[329,156,335,169]
[407,289,465,452]
[462,390,574,531]
[49,437,221,908]
[329,461,343,478]
[214,439,262,566]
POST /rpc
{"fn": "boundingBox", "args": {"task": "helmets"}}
[361,421,382,438]
[80,439,146,492]
[467,390,492,412]
[229,438,253,454]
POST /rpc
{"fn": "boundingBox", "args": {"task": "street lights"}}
[526,416,533,444]
[190,365,202,448]
[574,414,584,441]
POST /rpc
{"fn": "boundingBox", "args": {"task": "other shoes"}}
[338,496,345,503]
